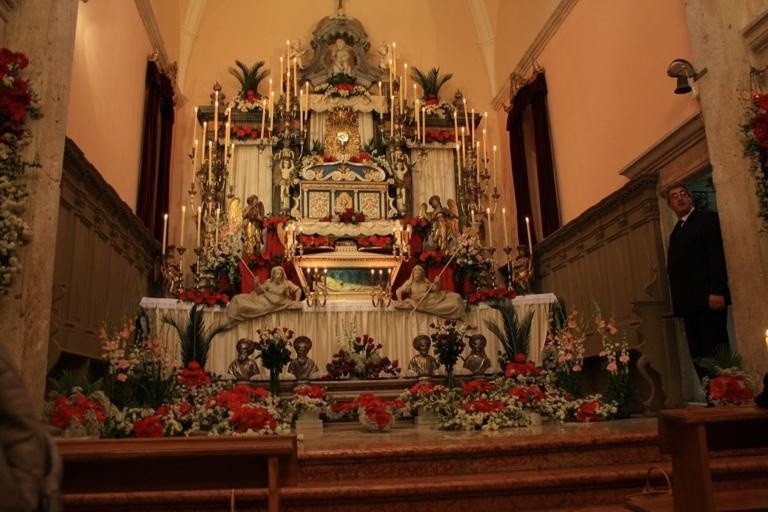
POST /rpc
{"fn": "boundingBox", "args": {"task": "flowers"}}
[285,382,331,411]
[430,320,467,364]
[320,322,401,378]
[97,292,237,385]
[41,383,291,440]
[463,287,630,392]
[326,381,619,431]
[256,326,295,367]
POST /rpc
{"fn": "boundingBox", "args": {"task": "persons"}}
[218,265,302,322]
[1,346,62,511]
[420,195,458,252]
[287,336,318,382]
[394,264,466,321]
[227,337,259,382]
[512,244,531,295]
[330,37,354,76]
[665,182,730,408]
[162,244,181,297]
[463,335,492,374]
[240,194,267,249]
[405,335,440,378]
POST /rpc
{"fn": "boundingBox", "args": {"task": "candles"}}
[284,224,303,259]
[191,90,229,237]
[371,266,394,307]
[306,266,328,308]
[261,37,310,157]
[467,205,534,294]
[391,225,412,256]
[453,96,498,234]
[159,206,222,298]
[375,41,427,180]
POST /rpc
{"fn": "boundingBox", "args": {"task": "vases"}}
[444,365,456,387]
[267,368,282,394]
[294,412,324,439]
